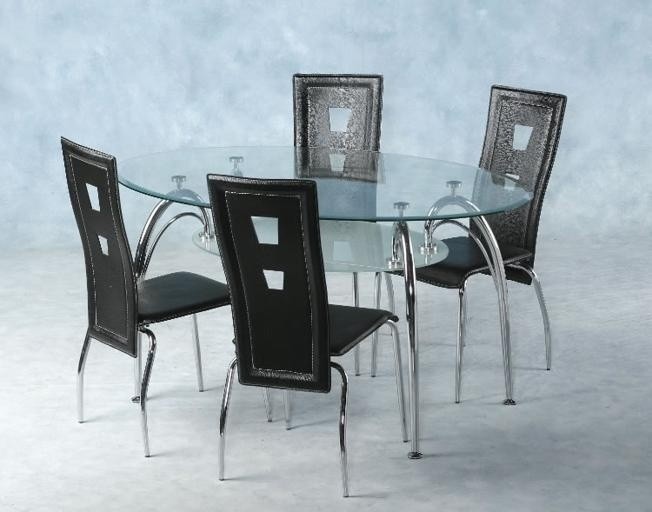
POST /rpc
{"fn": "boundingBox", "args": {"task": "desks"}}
[108,144,535,466]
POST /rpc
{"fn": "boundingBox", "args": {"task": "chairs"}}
[206,173,409,494]
[59,135,274,456]
[373,82,570,404]
[290,73,397,371]
